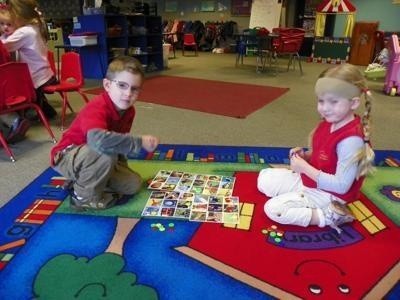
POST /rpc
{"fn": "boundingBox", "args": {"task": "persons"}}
[49,55,160,211]
[0,8,16,41]
[0,1,60,122]
[0,39,30,143]
[256,62,375,236]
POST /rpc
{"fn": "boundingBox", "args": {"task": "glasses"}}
[104,76,144,94]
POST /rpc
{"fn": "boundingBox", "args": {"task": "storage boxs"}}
[67,33,100,47]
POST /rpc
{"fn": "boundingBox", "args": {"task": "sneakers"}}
[322,200,357,227]
[71,191,118,209]
[112,192,124,203]
[7,116,29,143]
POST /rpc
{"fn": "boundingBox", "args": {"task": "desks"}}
[54,44,105,83]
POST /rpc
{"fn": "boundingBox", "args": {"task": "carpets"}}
[0,144,400,300]
[78,73,291,120]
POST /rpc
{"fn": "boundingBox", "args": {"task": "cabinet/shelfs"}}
[70,13,166,78]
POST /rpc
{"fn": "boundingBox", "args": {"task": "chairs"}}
[0,40,89,162]
[162,32,183,59]
[231,24,305,77]
[181,33,200,56]
